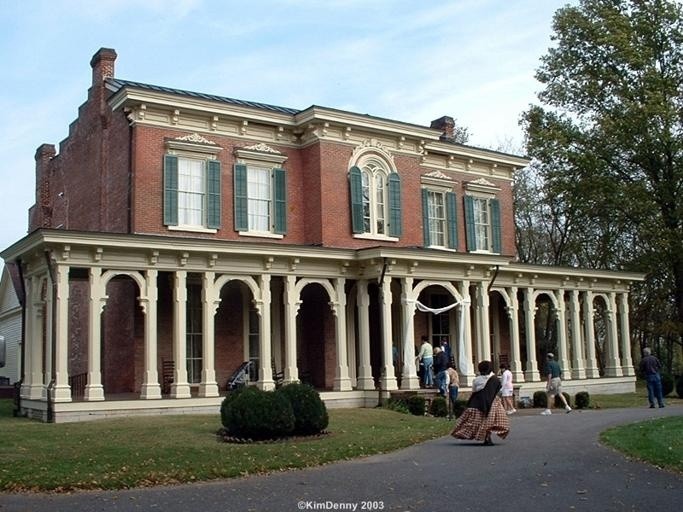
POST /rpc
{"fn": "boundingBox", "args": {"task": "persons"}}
[403,335,460,419]
[540,352,571,415]
[451,360,511,446]
[498,363,517,416]
[392,340,399,376]
[640,346,668,408]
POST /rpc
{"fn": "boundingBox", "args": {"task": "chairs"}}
[271,358,283,381]
[499,355,508,365]
[161,357,175,394]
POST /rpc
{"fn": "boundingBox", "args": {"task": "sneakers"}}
[505,409,516,415]
[565,407,572,413]
[540,411,552,416]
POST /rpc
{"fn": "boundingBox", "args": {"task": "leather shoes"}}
[649,406,655,408]
[659,406,664,408]
